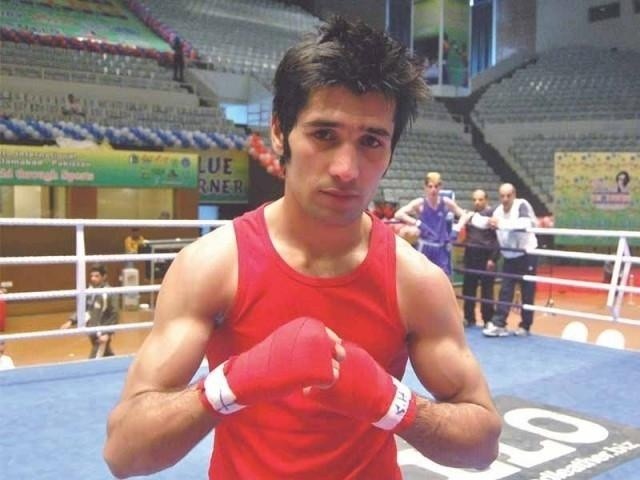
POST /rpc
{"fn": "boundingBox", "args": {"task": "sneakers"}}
[514,328,529,336]
[483,326,507,336]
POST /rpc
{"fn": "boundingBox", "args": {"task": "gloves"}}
[416,223,434,235]
[446,228,458,246]
[196,316,337,420]
[311,336,417,436]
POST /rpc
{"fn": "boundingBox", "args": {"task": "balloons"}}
[0,0,212,69]
[0,115,286,180]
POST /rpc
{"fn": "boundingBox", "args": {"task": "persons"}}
[60,93,89,117]
[170,35,185,82]
[61,263,121,359]
[418,31,466,85]
[123,227,145,268]
[0,339,15,370]
[395,173,540,337]
[101,20,502,480]
